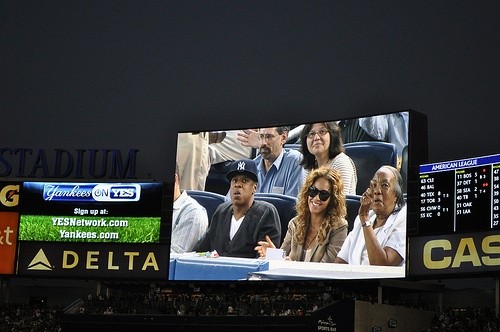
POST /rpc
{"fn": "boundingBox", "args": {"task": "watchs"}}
[361,221,372,227]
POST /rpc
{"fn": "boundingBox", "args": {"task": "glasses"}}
[258,134,278,141]
[308,186,332,201]
[305,128,330,138]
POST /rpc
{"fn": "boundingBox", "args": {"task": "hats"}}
[226,159,258,180]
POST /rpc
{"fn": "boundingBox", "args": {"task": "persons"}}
[224,126,308,200]
[176,112,409,191]
[254,166,348,263]
[0,281,500,331]
[170,162,208,253]
[334,165,407,266]
[192,159,282,259]
[299,121,358,195]
